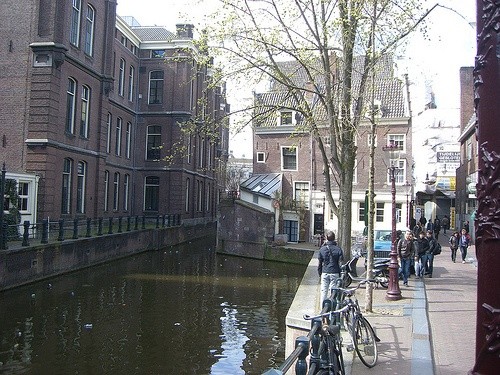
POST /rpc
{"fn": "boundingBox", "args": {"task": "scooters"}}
[340,248,403,289]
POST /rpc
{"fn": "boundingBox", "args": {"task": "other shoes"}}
[462,261,465,264]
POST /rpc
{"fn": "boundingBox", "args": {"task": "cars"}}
[374,230,409,251]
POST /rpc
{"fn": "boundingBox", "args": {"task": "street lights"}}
[380,139,402,301]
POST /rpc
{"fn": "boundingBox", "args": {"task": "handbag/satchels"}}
[432,240,441,255]
[415,262,419,276]
[338,266,341,277]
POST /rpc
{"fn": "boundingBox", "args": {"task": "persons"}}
[427,219,434,234]
[449,231,459,263]
[409,230,439,278]
[459,227,471,264]
[412,215,427,237]
[440,216,449,234]
[320,233,326,244]
[465,220,469,233]
[397,231,414,287]
[434,220,441,240]
[317,231,343,310]
[434,215,440,223]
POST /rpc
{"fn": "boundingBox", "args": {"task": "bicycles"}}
[303,258,381,375]
